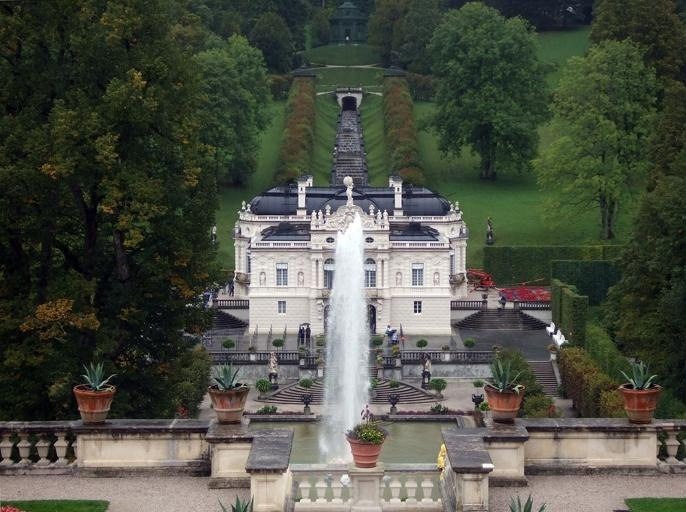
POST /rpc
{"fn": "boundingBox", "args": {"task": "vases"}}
[347,435,384,468]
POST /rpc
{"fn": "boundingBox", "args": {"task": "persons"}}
[306,325,311,338]
[318,209,323,223]
[382,209,388,220]
[222,280,234,297]
[343,176,354,202]
[498,297,502,309]
[312,210,317,222]
[385,325,398,345]
[300,327,305,338]
[369,205,375,215]
[326,205,331,215]
[501,296,506,310]
[376,210,382,220]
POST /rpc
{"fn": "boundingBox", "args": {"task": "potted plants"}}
[483,356,528,424]
[70,360,121,425]
[616,359,665,424]
[205,360,252,424]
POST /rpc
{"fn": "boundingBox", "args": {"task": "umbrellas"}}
[498,300,506,304]
[499,293,508,297]
[301,323,310,326]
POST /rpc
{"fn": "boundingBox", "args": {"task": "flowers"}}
[345,404,387,444]
